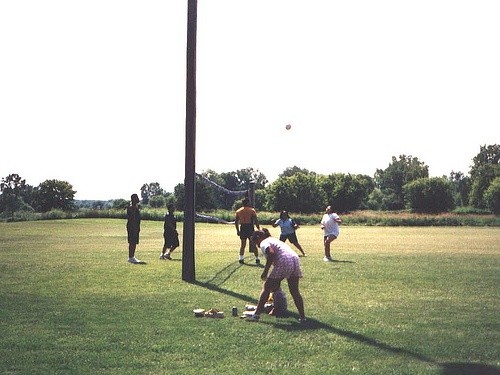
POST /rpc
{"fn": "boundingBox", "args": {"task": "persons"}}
[126,194,141,264]
[160,204,179,260]
[235,197,261,264]
[245,228,307,322]
[320,205,342,262]
[243,281,287,315]
[271,208,308,257]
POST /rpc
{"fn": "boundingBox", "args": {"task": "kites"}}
[286,124,291,130]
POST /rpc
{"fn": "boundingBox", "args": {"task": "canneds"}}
[232,307,237,316]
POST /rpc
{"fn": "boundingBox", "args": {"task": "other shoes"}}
[244,314,260,323]
[256,259,260,263]
[239,259,244,264]
[128,257,140,263]
[160,255,164,260]
[164,253,172,260]
[323,255,332,262]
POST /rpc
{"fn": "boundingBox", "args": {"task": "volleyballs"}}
[285,124,291,130]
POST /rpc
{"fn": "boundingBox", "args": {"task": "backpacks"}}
[265,285,288,315]
